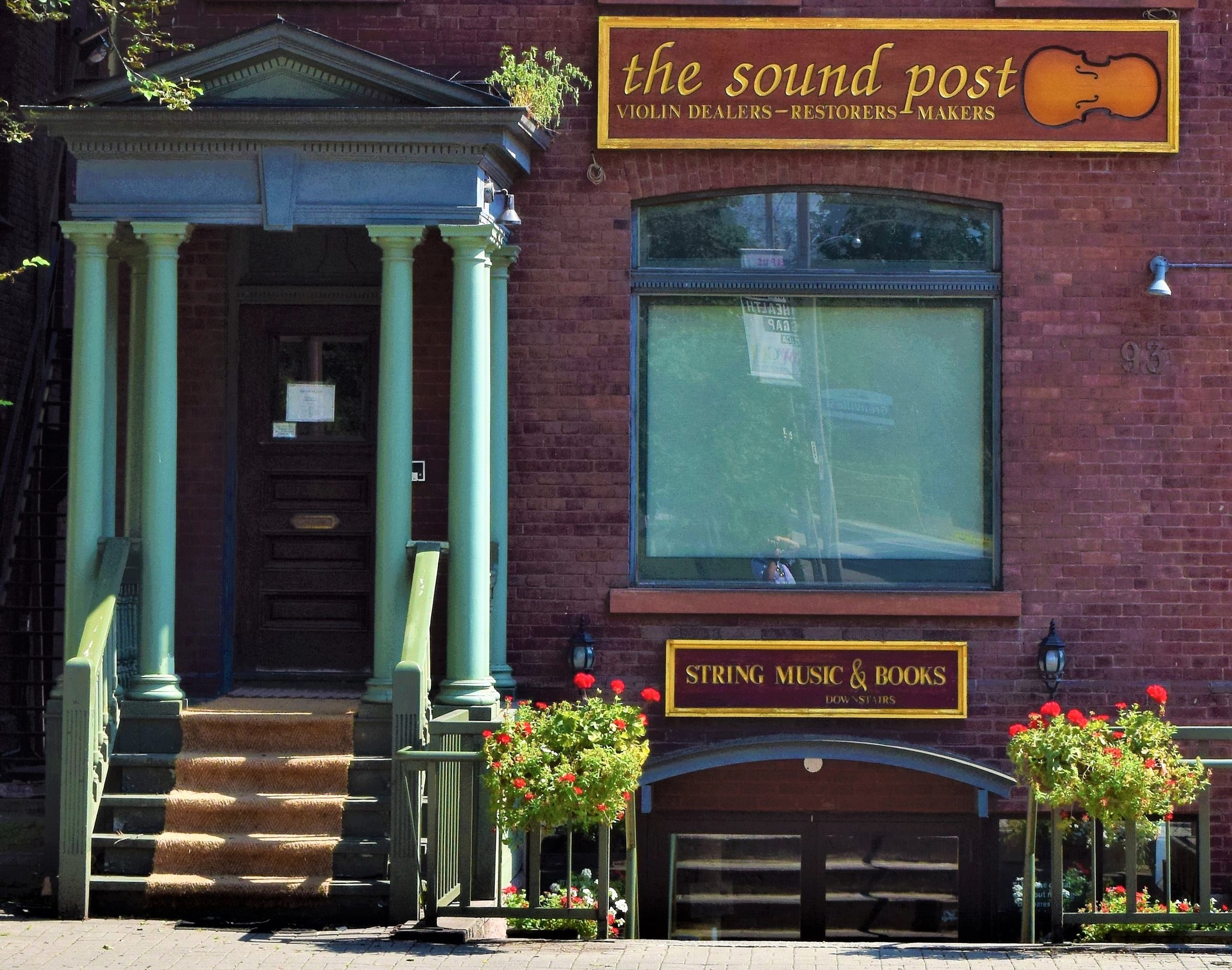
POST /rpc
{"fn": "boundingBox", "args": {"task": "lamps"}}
[566,613,597,705]
[1036,618,1068,701]
[1147,255,1172,295]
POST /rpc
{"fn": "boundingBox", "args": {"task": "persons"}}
[749,534,801,585]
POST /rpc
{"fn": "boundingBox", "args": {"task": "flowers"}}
[1078,883,1228,943]
[477,672,663,852]
[1012,876,1042,907]
[499,867,626,940]
[1063,861,1089,913]
[1006,677,1215,846]
